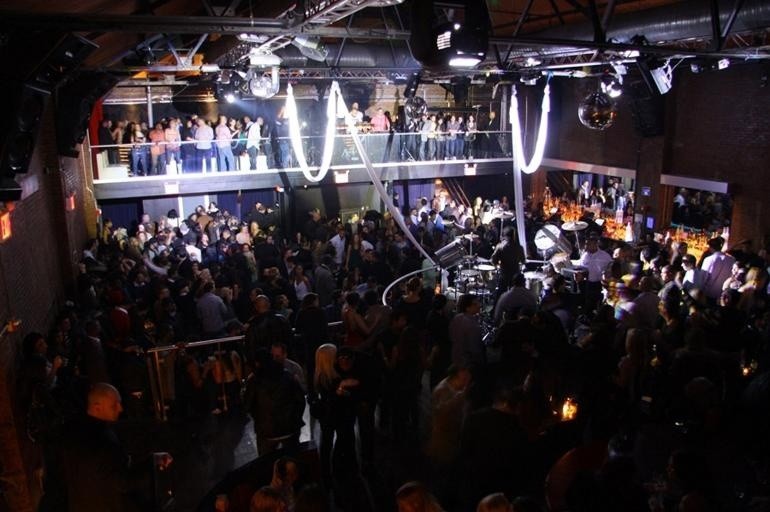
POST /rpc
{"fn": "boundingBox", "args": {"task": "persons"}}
[1,177,769,512]
[346,103,499,162]
[100,113,293,177]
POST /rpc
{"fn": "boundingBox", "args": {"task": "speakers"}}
[636,63,673,102]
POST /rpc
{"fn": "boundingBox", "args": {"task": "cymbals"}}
[464,234,479,239]
[493,212,514,219]
[463,255,489,262]
[561,220,588,231]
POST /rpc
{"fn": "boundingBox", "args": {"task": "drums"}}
[473,264,495,281]
[560,265,589,288]
[461,269,479,282]
[522,260,548,280]
[550,252,573,274]
[534,224,572,256]
